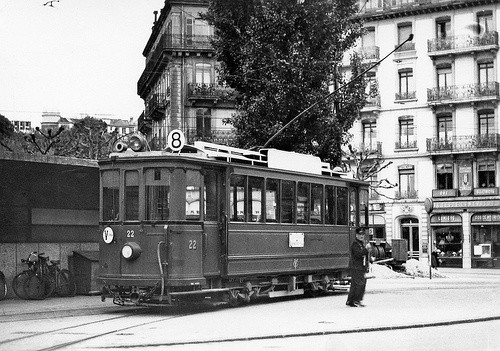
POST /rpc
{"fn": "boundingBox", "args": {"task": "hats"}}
[355,227,366,235]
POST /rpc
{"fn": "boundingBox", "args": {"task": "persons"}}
[345,227,372,308]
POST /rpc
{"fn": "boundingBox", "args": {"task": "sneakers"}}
[346,300,357,307]
[355,300,365,307]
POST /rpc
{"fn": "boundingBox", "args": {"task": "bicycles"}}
[0,270,8,301]
[23,252,78,299]
[12,252,69,300]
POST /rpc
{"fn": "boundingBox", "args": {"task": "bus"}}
[98,129,369,306]
